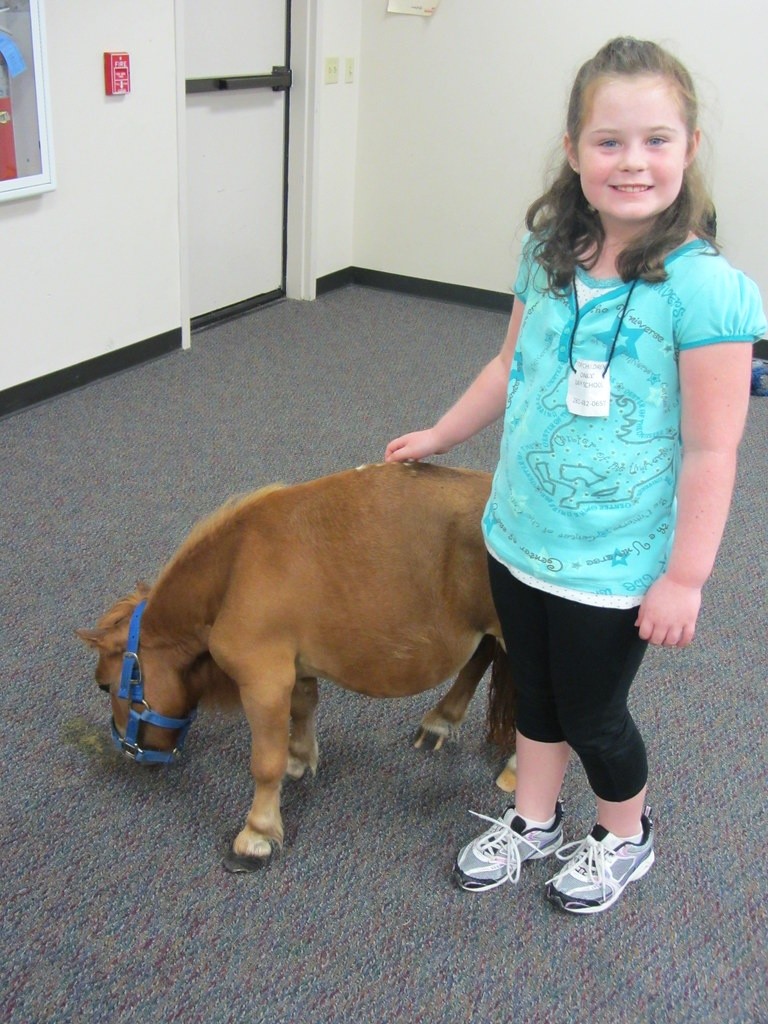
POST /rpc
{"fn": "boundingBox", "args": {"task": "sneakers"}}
[545,806,656,914]
[451,799,565,892]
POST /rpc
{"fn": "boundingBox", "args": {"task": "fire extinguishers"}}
[0,26,17,182]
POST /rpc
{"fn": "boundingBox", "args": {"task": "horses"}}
[72,458,520,873]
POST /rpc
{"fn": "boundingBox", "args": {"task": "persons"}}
[384,37,767,914]
